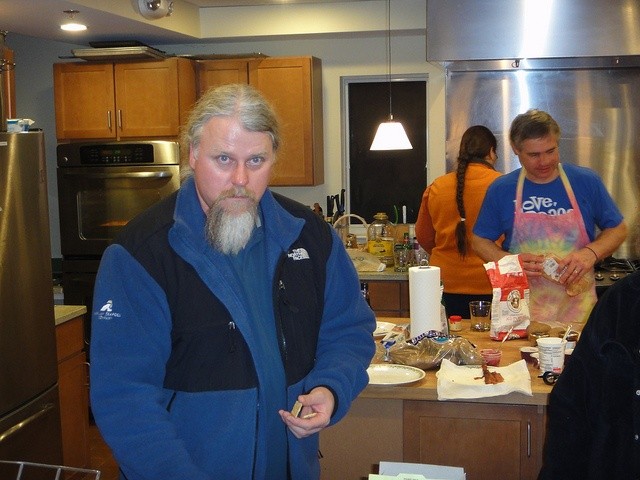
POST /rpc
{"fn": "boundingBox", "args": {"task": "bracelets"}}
[584,246,598,263]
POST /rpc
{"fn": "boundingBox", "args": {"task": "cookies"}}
[291,401,300,418]
[303,413,316,419]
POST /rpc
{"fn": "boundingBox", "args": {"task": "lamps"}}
[369,114,414,152]
[138,0,176,20]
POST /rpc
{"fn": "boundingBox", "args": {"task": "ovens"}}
[55,139,181,260]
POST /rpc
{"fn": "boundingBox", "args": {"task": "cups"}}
[535,337,568,377]
[469,301,491,332]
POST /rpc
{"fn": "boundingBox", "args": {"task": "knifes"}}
[327,196,331,217]
[331,195,335,216]
[334,194,340,215]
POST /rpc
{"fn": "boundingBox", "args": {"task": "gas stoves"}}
[595,256,640,287]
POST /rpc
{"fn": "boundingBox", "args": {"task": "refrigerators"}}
[0,130,65,479]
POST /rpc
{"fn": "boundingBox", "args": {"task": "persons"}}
[470,108,628,324]
[538,268,638,479]
[415,125,506,318]
[89,84,376,478]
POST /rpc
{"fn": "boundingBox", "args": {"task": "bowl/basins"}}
[479,348,503,366]
[520,347,538,361]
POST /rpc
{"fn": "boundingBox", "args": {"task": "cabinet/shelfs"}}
[1,48,17,132]
[53,55,196,144]
[361,280,401,318]
[193,56,325,187]
[402,398,547,479]
[402,280,410,318]
[55,314,92,480]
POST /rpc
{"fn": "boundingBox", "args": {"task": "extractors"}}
[425,1,639,71]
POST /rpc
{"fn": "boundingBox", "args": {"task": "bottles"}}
[402,232,411,264]
[411,237,420,266]
[394,243,408,273]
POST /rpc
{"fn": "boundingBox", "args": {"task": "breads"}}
[536,253,594,297]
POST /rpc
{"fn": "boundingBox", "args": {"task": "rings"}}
[574,269,578,273]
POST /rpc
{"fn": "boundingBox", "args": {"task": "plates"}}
[367,364,426,386]
[373,321,396,337]
